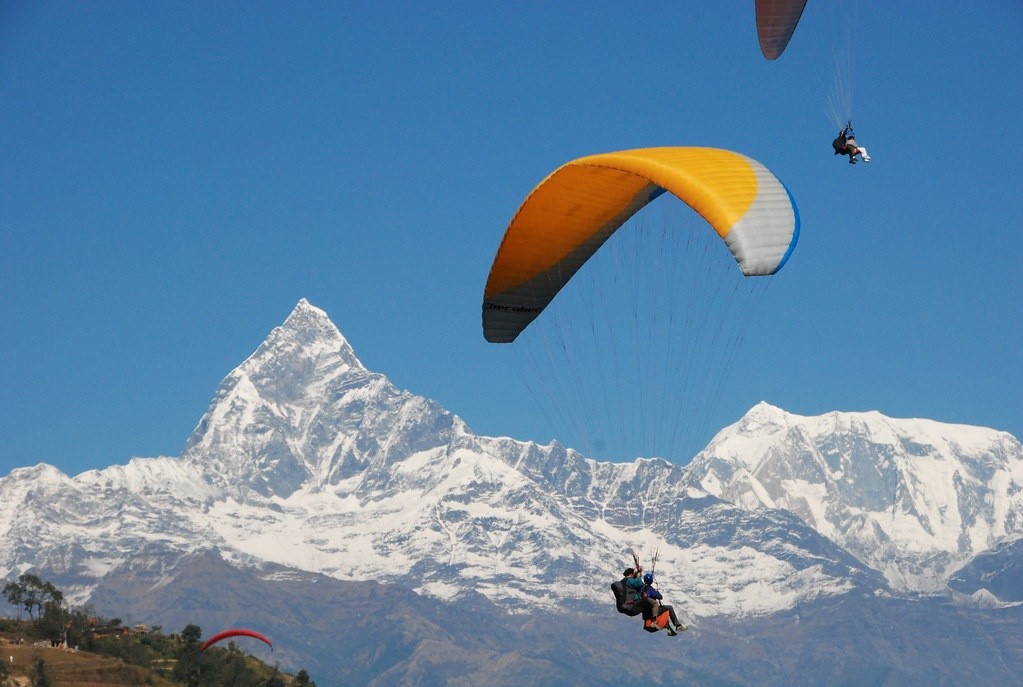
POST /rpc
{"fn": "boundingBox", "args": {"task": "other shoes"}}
[676,624,688,631]
[667,630,677,636]
[651,621,663,630]
[851,157,858,161]
[863,156,870,159]
[849,160,856,164]
[864,159,870,162]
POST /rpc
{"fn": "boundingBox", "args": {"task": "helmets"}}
[644,574,653,584]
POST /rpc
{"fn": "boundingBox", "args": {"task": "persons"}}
[641,574,688,637]
[619,564,662,633]
[837,122,859,164]
[846,135,871,162]
